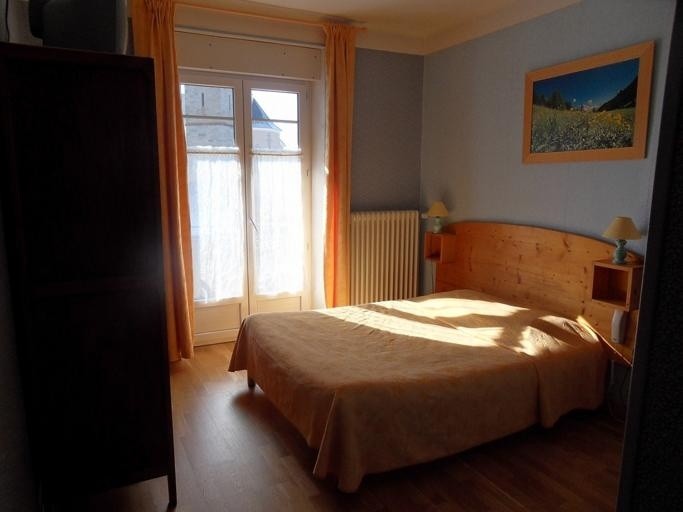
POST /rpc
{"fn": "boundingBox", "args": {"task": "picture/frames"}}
[522,40,655,165]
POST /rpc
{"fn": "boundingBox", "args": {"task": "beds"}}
[227,222,641,492]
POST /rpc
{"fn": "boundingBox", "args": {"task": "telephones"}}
[610,308,627,344]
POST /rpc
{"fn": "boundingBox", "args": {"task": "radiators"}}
[347,210,426,307]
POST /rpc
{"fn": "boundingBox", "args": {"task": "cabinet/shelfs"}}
[1,42,176,511]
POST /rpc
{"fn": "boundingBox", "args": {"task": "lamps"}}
[602,217,641,265]
[427,201,449,234]
